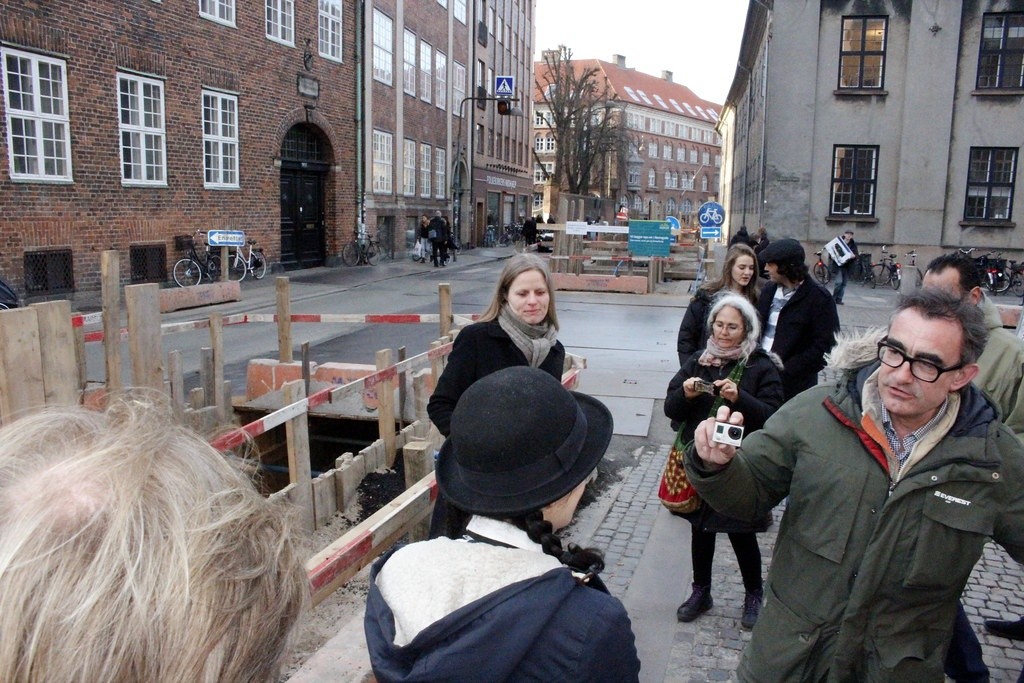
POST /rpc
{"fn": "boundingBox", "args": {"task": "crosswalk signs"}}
[495,75,516,93]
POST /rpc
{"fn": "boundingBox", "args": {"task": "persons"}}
[758,228,769,249]
[663,292,784,629]
[680,286,1024,683]
[746,232,761,255]
[828,230,858,305]
[677,244,761,367]
[364,366,641,683]
[0,385,314,683]
[521,214,555,247]
[427,252,566,540]
[756,239,840,404]
[418,210,458,267]
[731,226,749,248]
[921,250,1024,445]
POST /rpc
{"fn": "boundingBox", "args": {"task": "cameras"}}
[713,422,744,447]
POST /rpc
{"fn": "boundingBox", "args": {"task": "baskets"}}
[174,234,193,251]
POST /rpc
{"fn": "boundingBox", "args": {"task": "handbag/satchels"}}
[412,239,422,260]
[658,432,701,513]
[429,229,437,238]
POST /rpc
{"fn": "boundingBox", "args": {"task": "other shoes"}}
[984,614,1024,641]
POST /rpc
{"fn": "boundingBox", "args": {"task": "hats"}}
[436,366,614,516]
[845,229,854,235]
[757,238,805,263]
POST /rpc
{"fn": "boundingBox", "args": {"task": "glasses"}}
[877,335,961,383]
[712,322,745,333]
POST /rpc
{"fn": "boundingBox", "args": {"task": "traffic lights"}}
[497,100,511,115]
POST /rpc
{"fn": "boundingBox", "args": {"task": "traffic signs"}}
[206,230,245,247]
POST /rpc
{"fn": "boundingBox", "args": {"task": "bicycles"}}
[814,245,831,289]
[957,247,1024,298]
[850,250,876,291]
[343,229,382,266]
[871,243,903,291]
[484,222,525,248]
[903,248,924,287]
[173,228,225,288]
[228,239,269,282]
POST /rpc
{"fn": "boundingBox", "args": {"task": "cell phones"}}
[694,380,720,396]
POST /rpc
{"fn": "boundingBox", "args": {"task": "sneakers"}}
[677,582,713,621]
[741,593,762,628]
